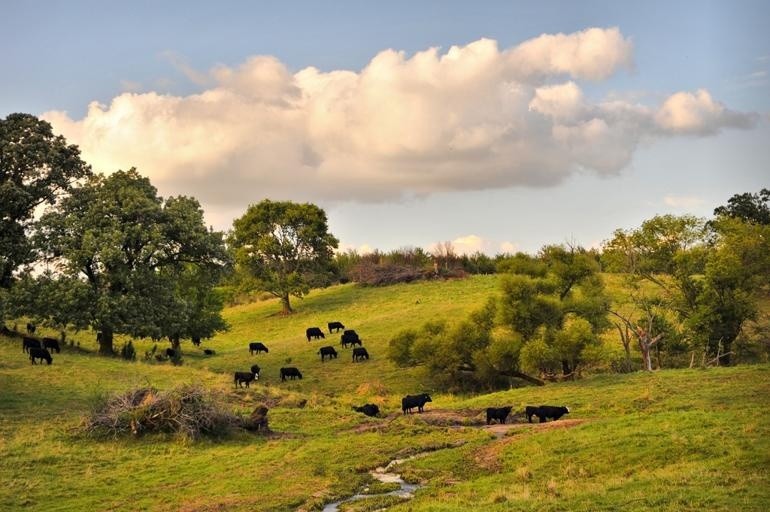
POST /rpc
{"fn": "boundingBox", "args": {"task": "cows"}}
[526,406,546,422]
[23,337,60,365]
[402,393,428,416]
[306,322,369,363]
[353,406,363,412]
[539,406,570,422]
[280,367,302,381]
[407,394,432,413]
[249,343,268,355]
[27,322,36,333]
[204,349,215,356]
[363,403,380,416]
[166,348,176,360]
[251,365,260,381]
[234,372,254,388]
[486,406,512,424]
